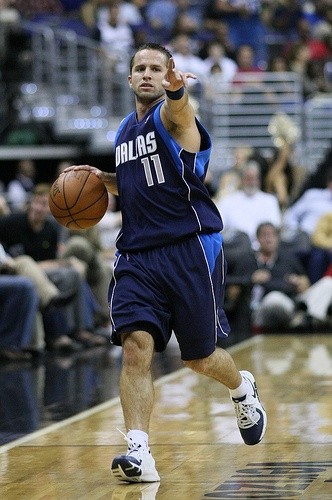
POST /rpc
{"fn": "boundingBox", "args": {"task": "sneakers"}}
[229,371,267,445]
[110,442,160,481]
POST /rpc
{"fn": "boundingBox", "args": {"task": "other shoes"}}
[41,288,76,315]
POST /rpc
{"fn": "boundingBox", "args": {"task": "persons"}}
[0,8,54,146]
[96,0,332,138]
[63,43,268,483]
[6,160,35,214]
[204,137,332,348]
[0,340,120,444]
[0,184,122,353]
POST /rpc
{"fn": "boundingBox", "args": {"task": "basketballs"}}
[48,169,110,230]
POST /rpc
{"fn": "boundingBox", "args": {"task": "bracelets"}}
[165,88,185,100]
